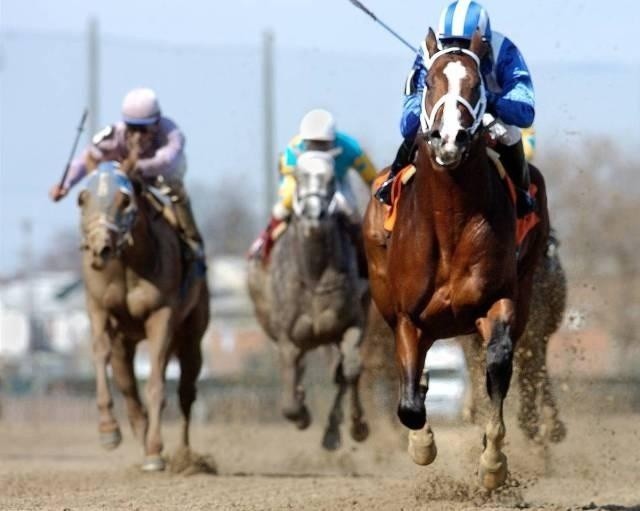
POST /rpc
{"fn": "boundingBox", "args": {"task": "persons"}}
[246,106,378,257]
[373,0,536,218]
[46,86,209,278]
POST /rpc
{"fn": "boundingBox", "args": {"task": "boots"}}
[497,141,534,219]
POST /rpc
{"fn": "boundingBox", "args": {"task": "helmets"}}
[437,0,493,41]
[122,88,160,124]
[298,110,333,141]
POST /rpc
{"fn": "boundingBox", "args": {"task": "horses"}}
[360,26,559,490]
[73,148,216,479]
[462,243,566,441]
[243,141,375,455]
